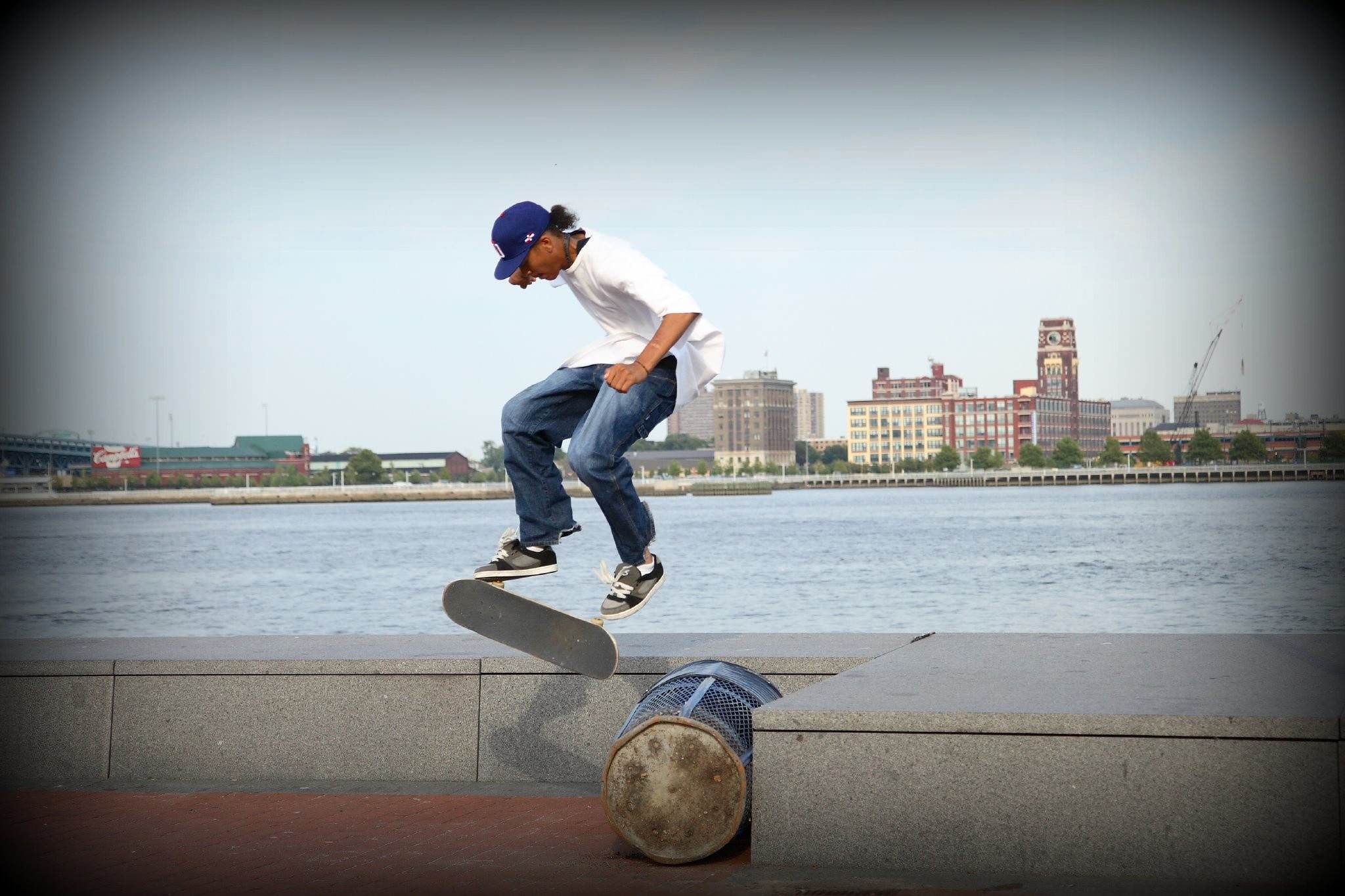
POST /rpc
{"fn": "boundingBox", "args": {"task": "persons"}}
[473,201,726,620]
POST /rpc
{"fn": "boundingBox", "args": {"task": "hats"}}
[491,201,550,280]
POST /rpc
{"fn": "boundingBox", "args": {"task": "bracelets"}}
[635,361,649,375]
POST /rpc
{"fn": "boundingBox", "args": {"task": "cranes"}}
[1167,328,1223,450]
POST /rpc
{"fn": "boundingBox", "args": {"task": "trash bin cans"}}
[600,658,786,865]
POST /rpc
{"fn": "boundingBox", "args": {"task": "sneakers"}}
[473,524,558,583]
[592,554,666,620]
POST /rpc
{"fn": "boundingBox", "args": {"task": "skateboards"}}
[441,578,619,682]
